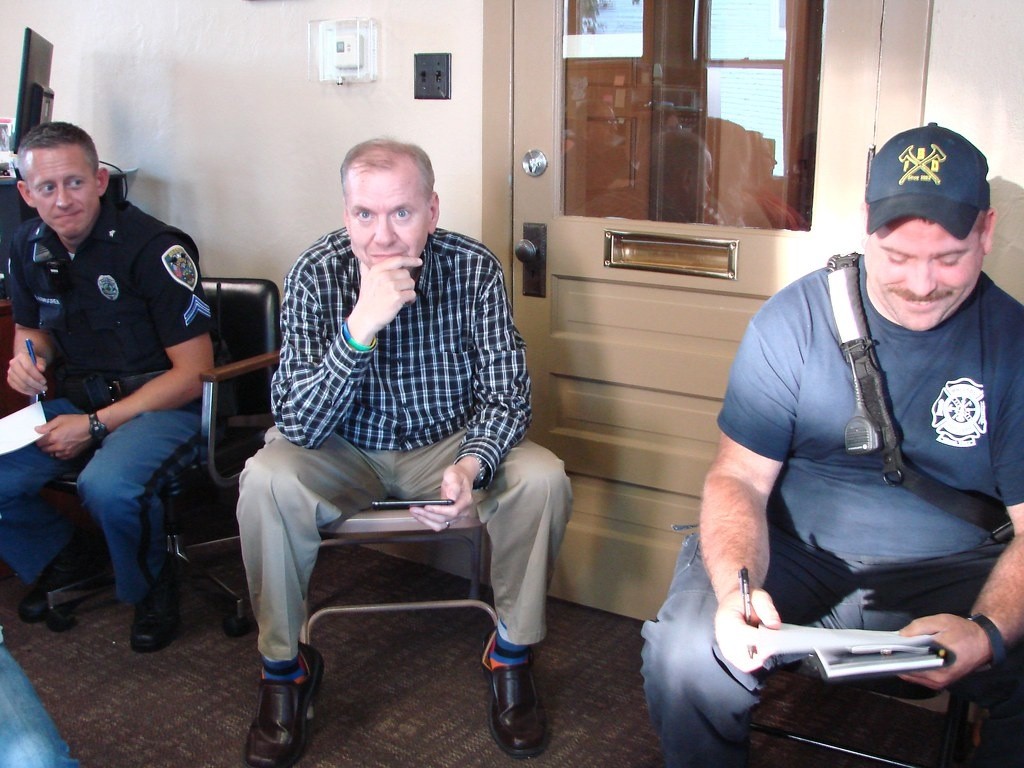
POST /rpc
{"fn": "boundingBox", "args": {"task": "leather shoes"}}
[480,624,548,760]
[244,639,324,768]
[17,541,108,622]
[129,550,179,653]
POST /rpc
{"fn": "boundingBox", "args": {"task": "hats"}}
[864,122,991,244]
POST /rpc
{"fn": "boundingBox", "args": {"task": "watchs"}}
[470,453,491,489]
[88,411,108,442]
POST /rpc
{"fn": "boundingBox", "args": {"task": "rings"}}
[446,521,451,529]
[53,452,60,459]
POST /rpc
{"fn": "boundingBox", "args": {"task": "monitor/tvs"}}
[10,24,54,152]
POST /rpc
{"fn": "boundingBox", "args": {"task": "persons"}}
[236,139,572,768]
[0,121,218,653]
[0,645,81,768]
[641,123,1024,768]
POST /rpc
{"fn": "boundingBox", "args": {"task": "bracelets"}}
[343,316,377,352]
[968,613,1006,672]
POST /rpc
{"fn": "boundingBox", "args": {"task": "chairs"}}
[41,281,283,635]
[746,662,987,768]
[298,508,494,719]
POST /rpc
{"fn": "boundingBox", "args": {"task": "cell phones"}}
[370,497,452,508]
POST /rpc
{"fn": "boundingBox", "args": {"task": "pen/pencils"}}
[739,565,754,659]
[931,640,945,656]
[25,337,47,398]
